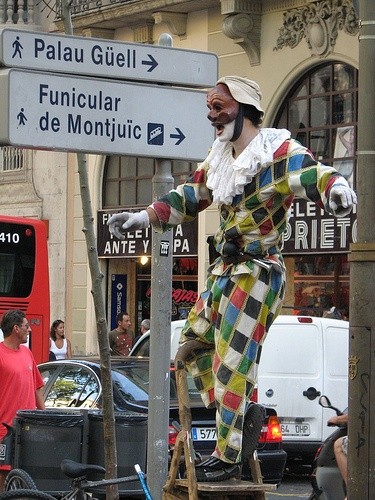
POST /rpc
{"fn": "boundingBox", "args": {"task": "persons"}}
[0,310,46,440]
[105,72,359,480]
[325,411,350,499]
[49,320,72,360]
[110,313,134,357]
[134,319,151,346]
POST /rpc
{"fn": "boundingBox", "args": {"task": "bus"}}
[1,213,50,365]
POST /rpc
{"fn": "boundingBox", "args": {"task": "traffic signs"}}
[0,28,219,88]
[0,67,217,162]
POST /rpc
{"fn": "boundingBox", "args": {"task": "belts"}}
[220,245,277,266]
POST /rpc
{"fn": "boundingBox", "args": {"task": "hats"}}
[215,75,265,124]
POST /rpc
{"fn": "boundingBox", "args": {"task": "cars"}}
[36,357,287,489]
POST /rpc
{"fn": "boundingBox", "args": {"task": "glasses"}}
[17,323,29,329]
[54,320,61,327]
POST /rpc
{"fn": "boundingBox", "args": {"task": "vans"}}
[121,314,349,476]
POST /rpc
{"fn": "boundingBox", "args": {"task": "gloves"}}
[107,210,149,239]
[329,185,357,211]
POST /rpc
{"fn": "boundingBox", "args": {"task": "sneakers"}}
[184,456,240,482]
[241,403,265,457]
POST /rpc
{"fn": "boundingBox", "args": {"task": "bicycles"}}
[0,458,153,500]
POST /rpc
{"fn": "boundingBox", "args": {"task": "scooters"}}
[310,394,349,500]
